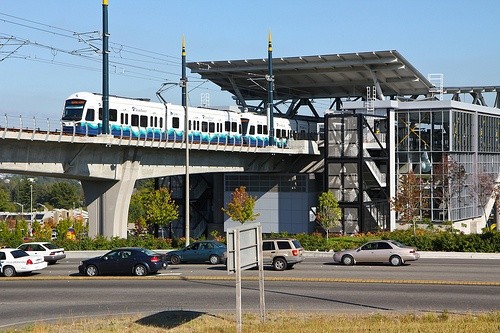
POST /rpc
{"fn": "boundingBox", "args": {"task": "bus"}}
[60,91,345,150]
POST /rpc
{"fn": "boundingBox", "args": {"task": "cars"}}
[78,247,168,277]
[16,241,67,264]
[0,248,48,277]
[333,240,420,266]
[166,241,227,265]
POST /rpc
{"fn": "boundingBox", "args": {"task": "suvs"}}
[261,238,304,271]
